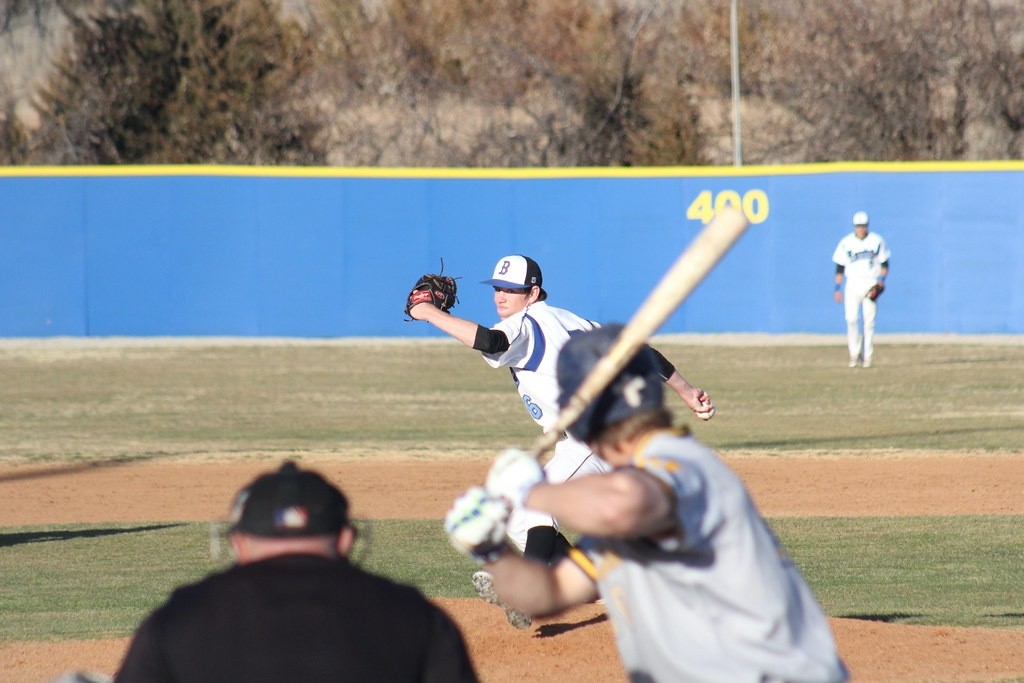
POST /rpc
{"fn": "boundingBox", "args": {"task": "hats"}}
[555,325,662,440]
[478,256,543,288]
[853,212,868,226]
[228,461,350,539]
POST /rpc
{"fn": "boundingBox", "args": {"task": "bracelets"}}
[877,275,886,281]
[835,284,841,290]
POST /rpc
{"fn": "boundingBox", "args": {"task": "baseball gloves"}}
[863,282,885,302]
[404,272,459,321]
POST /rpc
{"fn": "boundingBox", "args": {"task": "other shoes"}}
[861,358,872,368]
[848,357,858,367]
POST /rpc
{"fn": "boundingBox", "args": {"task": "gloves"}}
[485,449,547,508]
[443,488,513,564]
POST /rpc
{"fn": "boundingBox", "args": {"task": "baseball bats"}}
[450,204,752,557]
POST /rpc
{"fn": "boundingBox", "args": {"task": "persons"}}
[114,460,478,683]
[446,324,851,683]
[406,256,715,629]
[831,211,891,367]
